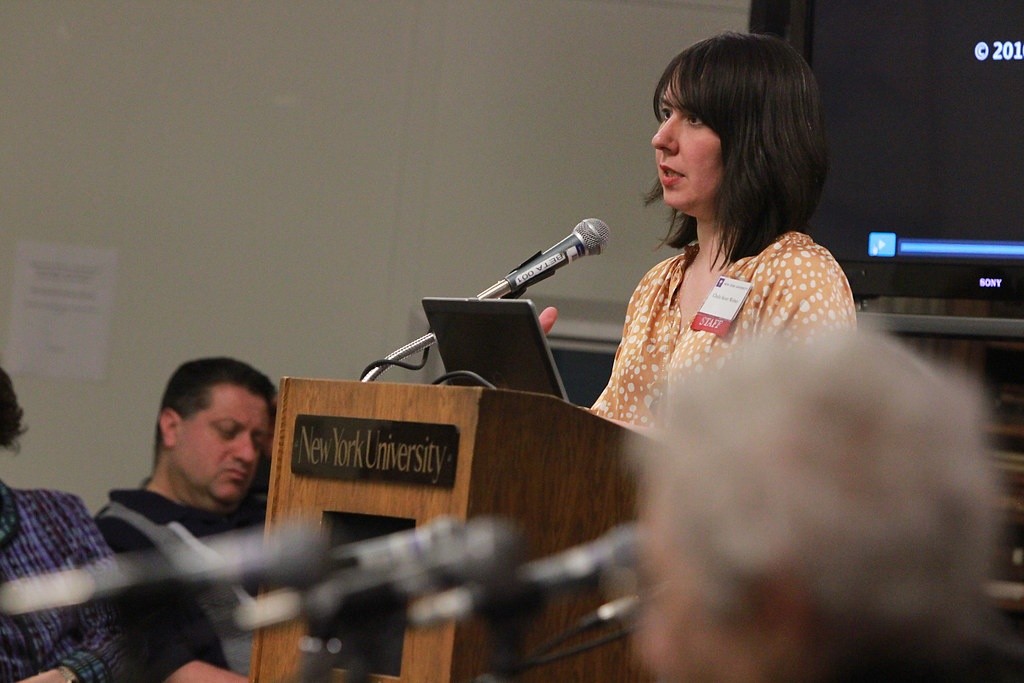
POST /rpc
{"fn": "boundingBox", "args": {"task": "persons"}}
[622,328,1024,683]
[537,33,856,441]
[0,367,131,683]
[94,355,278,683]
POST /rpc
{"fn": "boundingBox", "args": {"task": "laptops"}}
[420,296,571,405]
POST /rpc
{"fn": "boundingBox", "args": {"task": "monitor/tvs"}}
[786,0,1024,300]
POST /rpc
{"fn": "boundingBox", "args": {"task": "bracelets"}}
[57,666,79,683]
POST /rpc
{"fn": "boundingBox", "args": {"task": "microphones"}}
[0,514,651,627]
[476,219,610,300]
